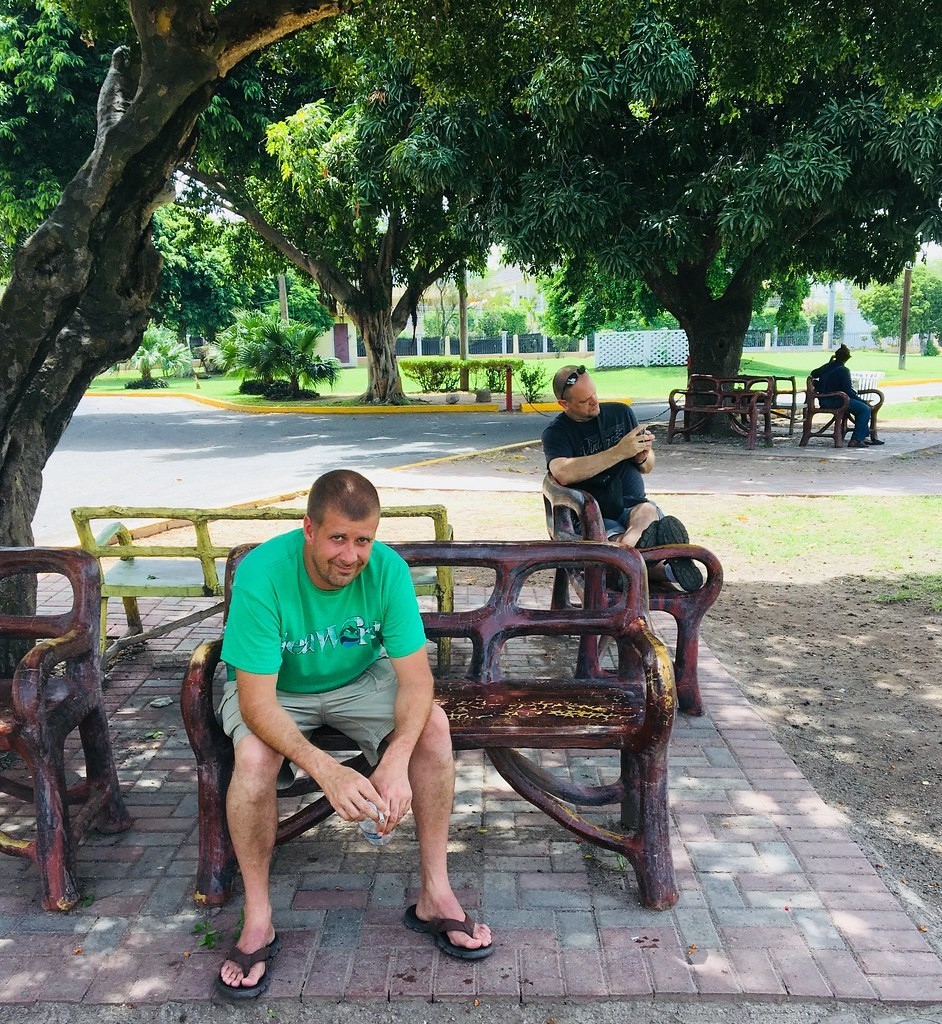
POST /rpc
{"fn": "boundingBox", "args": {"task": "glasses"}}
[560,365,587,399]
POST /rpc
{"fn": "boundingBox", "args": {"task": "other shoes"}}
[608,520,663,591]
[658,515,703,592]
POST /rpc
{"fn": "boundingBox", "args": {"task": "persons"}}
[811,343,873,448]
[217,469,495,999]
[540,365,704,594]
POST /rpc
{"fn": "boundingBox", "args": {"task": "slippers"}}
[215,931,282,999]
[847,441,870,448]
[403,904,494,960]
[865,438,884,445]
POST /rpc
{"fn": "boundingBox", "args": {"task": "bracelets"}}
[634,457,647,465]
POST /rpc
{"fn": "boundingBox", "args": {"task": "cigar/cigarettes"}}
[379,812,384,823]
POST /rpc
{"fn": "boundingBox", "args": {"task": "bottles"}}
[359,799,396,845]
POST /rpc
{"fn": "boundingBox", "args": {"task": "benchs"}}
[734,374,806,436]
[181,541,681,912]
[0,546,135,911]
[541,474,724,716]
[667,374,774,450]
[71,505,456,675]
[798,376,884,447]
